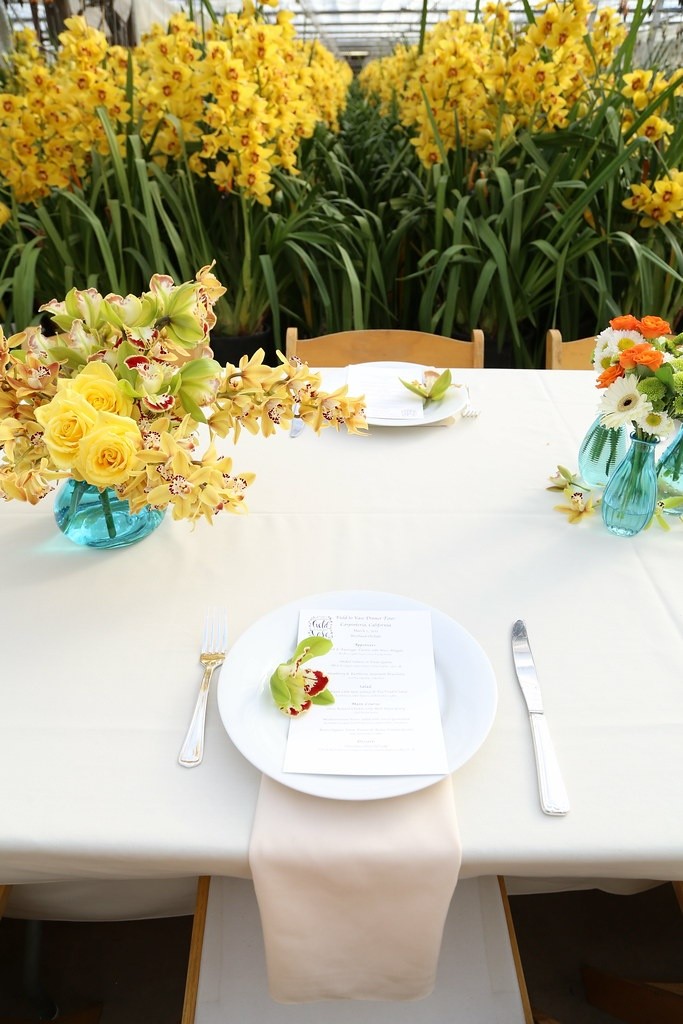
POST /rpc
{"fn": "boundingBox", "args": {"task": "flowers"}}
[591,314,682,443]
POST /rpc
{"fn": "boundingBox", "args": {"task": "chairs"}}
[546,329,597,371]
[286,327,484,368]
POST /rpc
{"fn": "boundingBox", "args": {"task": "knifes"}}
[510,620,566,815]
[290,404,305,437]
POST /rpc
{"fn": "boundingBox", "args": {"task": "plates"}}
[217,590,498,799]
[329,362,467,426]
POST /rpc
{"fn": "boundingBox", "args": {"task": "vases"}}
[603,436,662,536]
[656,426,683,518]
[53,474,169,549]
[0,260,370,532]
[578,415,629,490]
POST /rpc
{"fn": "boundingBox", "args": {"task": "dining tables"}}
[0,368,683,1024]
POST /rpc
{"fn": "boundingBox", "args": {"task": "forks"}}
[462,385,478,417]
[178,610,228,766]
